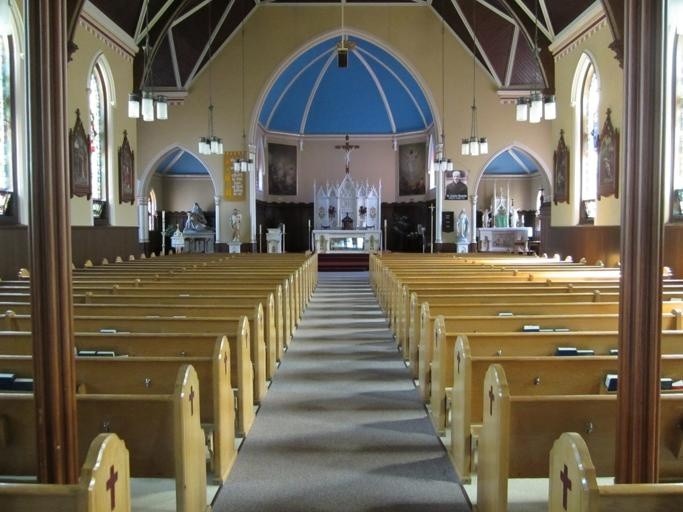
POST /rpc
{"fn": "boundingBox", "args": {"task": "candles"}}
[162,210,166,233]
[384,218,387,228]
[308,219,310,227]
[482,216,484,223]
[260,223,262,234]
[522,215,524,224]
[283,224,285,233]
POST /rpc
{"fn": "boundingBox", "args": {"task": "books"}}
[0,373,14,392]
[99,330,116,333]
[96,351,115,357]
[78,350,96,356]
[13,378,34,392]
[498,310,683,392]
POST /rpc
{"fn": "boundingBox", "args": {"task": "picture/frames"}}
[69,108,92,201]
[397,142,428,198]
[118,129,135,206]
[553,129,569,204]
[267,142,298,198]
[597,108,620,201]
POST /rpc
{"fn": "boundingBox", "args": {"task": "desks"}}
[312,230,380,254]
[478,227,528,251]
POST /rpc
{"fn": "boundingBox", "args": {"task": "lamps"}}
[338,0,348,68]
[433,0,557,173]
[128,0,254,173]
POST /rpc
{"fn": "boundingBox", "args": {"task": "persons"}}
[445,170,468,201]
[479,204,493,228]
[509,206,521,227]
[339,145,356,168]
[456,207,470,238]
[228,208,241,242]
[185,201,208,230]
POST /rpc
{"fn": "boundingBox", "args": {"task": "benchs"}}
[0,251,319,512]
[369,249,683,512]
